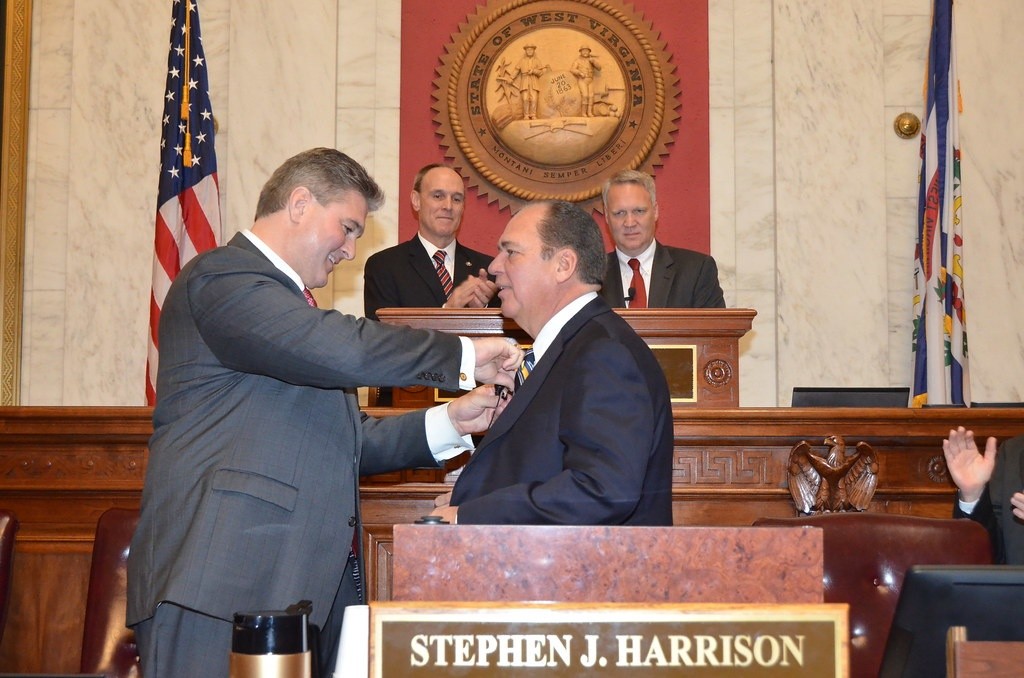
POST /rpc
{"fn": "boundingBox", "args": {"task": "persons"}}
[362,162,503,322]
[428,199,675,526]
[939,424,1024,566]
[123,143,523,678]
[597,168,726,307]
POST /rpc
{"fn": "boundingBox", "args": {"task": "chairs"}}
[753,507,990,676]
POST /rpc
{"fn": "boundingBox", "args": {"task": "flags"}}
[144,0,226,407]
[905,0,973,408]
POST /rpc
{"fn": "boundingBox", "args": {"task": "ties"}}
[628,258,647,309]
[513,346,534,394]
[302,287,317,309]
[432,251,453,302]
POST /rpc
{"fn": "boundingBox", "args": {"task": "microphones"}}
[624,287,635,301]
[494,384,508,395]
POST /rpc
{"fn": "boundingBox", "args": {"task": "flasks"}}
[230,609,311,678]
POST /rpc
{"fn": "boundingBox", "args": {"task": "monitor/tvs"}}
[879,564,1024,678]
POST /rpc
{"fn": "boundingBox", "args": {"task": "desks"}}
[376,302,755,424]
